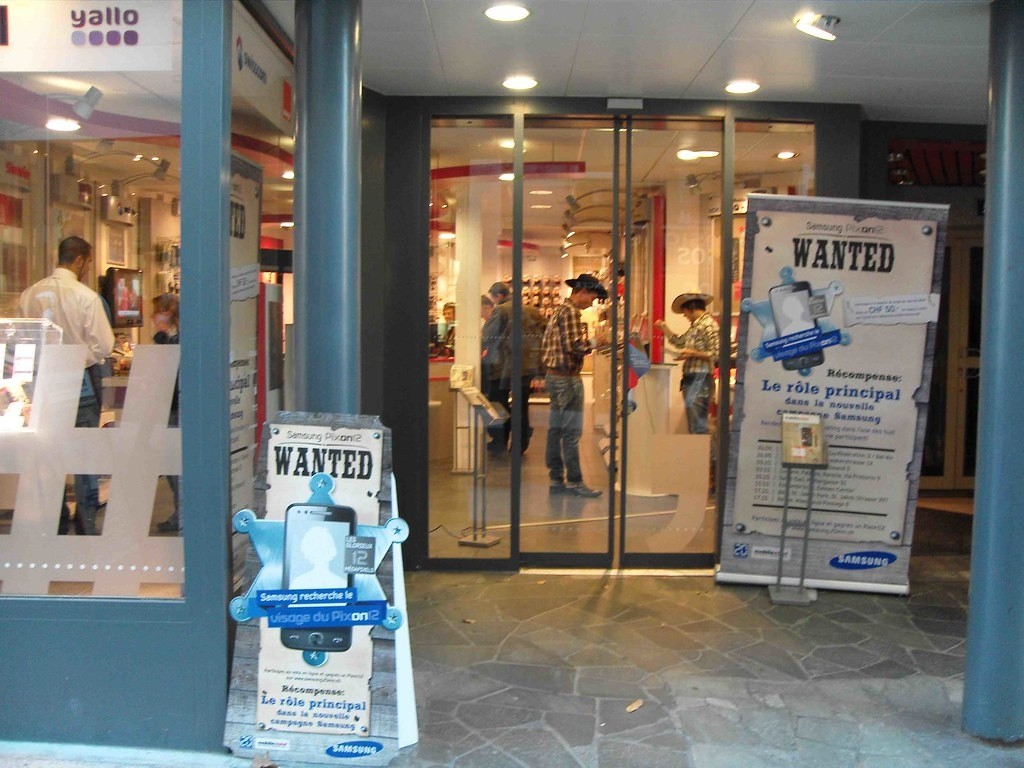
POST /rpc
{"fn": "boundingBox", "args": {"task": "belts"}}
[547,368,580,377]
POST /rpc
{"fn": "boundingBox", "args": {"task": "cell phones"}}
[279,503,358,653]
[769,281,825,371]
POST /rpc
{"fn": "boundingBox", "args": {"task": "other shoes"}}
[521,427,534,453]
[488,442,508,450]
[565,482,604,498]
[549,478,567,494]
[156,521,179,532]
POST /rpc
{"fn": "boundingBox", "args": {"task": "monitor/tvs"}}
[106,267,143,327]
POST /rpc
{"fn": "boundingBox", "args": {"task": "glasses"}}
[152,308,169,317]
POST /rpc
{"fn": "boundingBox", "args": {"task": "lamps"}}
[559,188,650,258]
[66,150,171,181]
[44,86,103,118]
[688,170,722,187]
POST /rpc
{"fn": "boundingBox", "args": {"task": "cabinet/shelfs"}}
[591,262,623,360]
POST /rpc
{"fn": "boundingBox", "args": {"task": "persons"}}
[481,281,547,453]
[480,295,502,401]
[443,302,455,351]
[151,294,180,532]
[540,274,607,497]
[654,293,718,433]
[17,234,115,534]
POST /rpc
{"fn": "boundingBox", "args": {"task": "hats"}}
[671,287,714,314]
[565,274,609,300]
